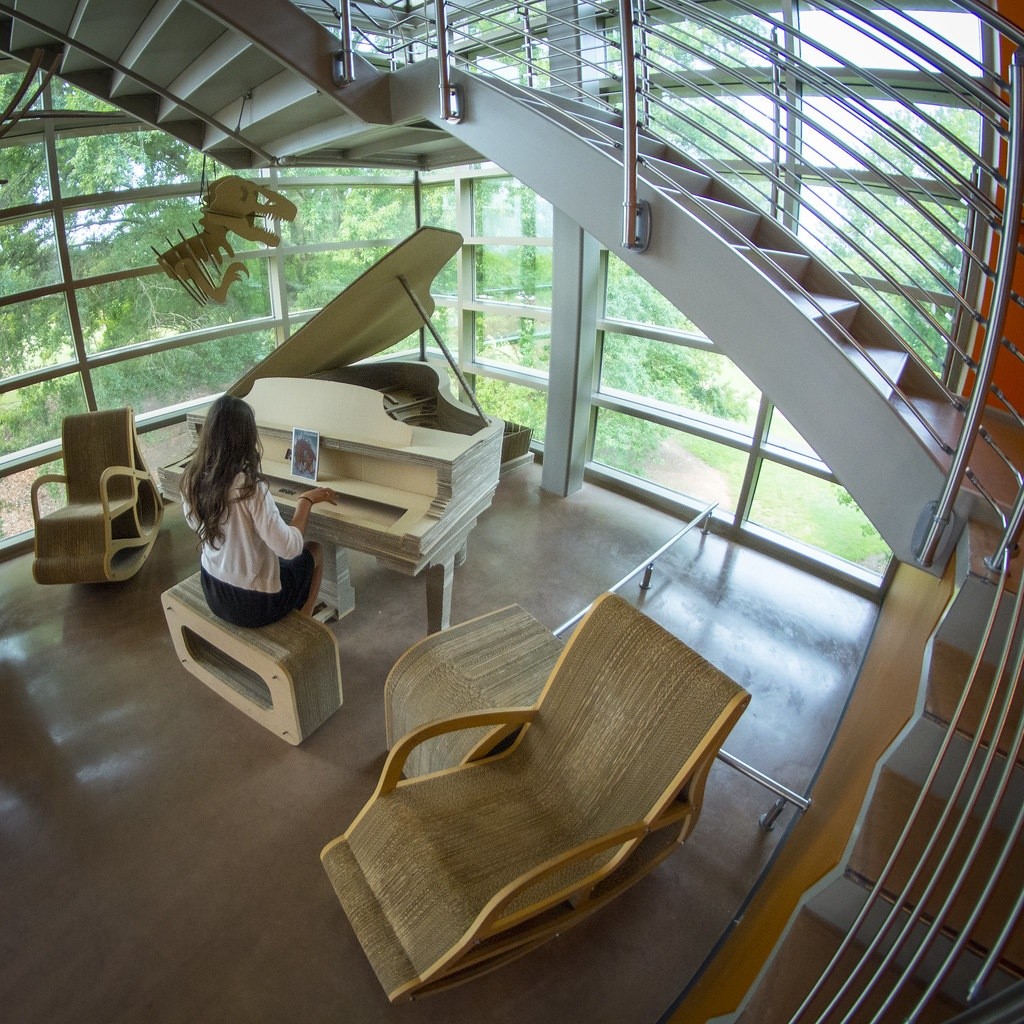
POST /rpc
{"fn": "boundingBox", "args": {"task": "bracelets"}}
[299,497,314,507]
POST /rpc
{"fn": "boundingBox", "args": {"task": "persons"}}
[180,395,340,629]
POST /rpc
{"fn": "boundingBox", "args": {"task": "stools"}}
[156,566,345,746]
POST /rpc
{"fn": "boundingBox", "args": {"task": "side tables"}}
[380,602,569,785]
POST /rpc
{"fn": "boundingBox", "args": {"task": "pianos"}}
[157,224,506,639]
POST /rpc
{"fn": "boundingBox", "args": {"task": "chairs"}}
[25,403,170,584]
[314,591,752,1005]
